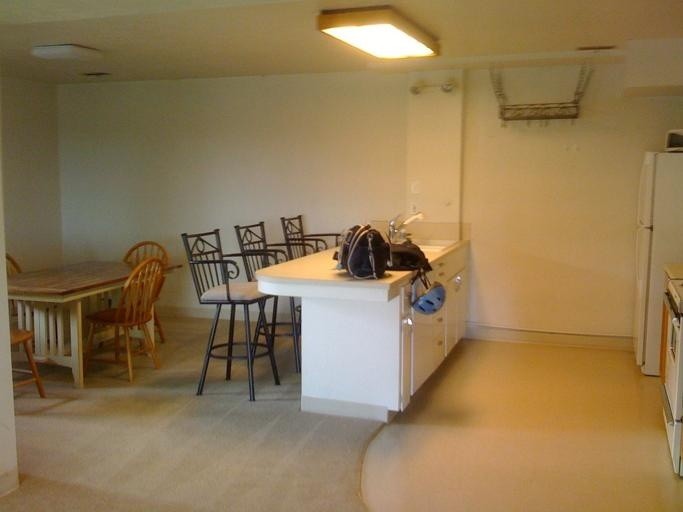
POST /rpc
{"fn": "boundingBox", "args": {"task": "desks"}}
[7,259,185,390]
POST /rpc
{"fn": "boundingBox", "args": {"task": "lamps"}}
[316,5,440,63]
[30,44,103,60]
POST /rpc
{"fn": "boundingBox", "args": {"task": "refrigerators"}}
[633,150,683,379]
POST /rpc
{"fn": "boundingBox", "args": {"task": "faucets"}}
[388,212,424,241]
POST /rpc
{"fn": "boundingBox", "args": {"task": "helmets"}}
[411,281,446,316]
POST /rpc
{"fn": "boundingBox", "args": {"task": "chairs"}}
[122,241,169,344]
[234,222,314,376]
[280,213,342,325]
[180,228,280,402]
[89,258,168,383]
[6,253,56,320]
[8,325,47,398]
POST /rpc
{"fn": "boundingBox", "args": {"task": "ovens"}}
[658,279,683,482]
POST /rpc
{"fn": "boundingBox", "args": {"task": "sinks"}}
[418,240,455,250]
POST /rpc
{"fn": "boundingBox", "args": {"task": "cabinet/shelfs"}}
[253,239,471,426]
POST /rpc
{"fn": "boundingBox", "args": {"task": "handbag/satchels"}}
[338,224,390,280]
[385,241,432,272]
